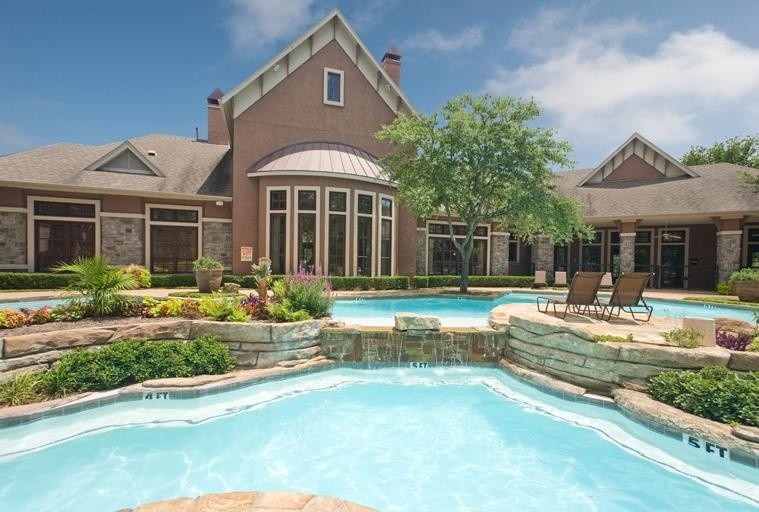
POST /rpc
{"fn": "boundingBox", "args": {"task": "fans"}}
[651,221,682,241]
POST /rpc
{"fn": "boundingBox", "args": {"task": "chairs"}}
[532,270,652,323]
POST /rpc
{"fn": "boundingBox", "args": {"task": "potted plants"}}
[729,269,759,301]
[248,260,271,303]
[193,257,224,293]
